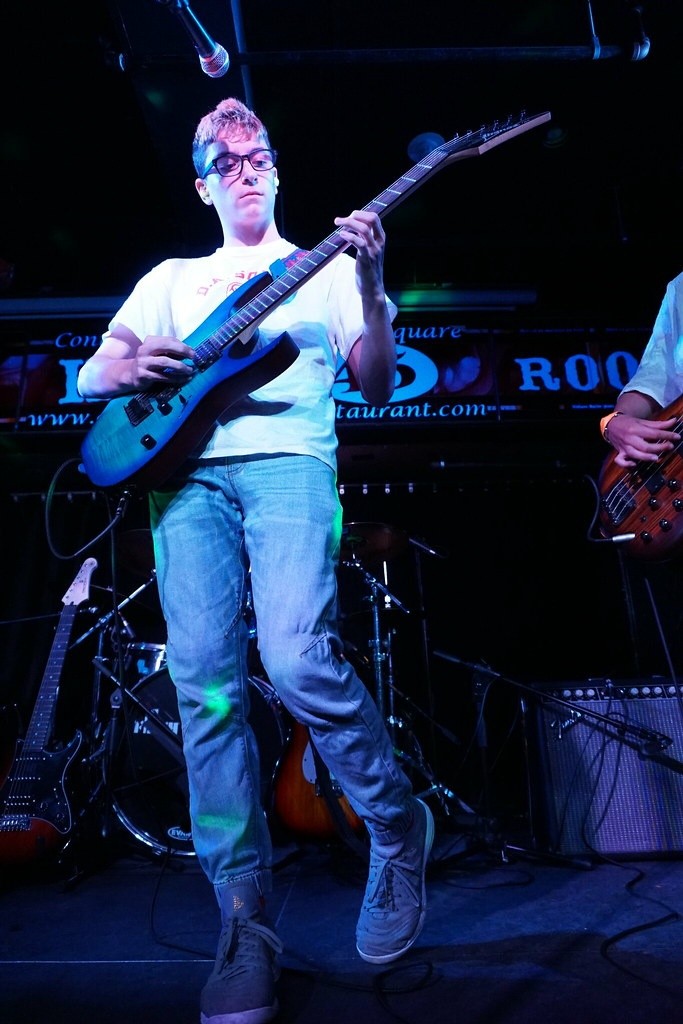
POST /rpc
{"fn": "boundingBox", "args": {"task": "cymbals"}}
[340,607,403,631]
[341,520,405,568]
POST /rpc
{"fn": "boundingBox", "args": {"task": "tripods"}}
[343,532,668,870]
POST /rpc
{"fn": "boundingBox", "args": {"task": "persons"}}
[76,99,435,1024]
[600,273,683,467]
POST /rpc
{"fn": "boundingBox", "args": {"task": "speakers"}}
[519,682,683,861]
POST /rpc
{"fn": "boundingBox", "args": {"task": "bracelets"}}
[600,411,620,442]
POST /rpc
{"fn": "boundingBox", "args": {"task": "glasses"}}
[202,149,279,177]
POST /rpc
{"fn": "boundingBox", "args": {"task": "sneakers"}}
[199,881,284,1024]
[355,798,435,964]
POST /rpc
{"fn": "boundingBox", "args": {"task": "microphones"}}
[639,737,673,756]
[167,0,230,78]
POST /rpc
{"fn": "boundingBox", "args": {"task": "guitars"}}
[593,394,682,556]
[81,108,552,489]
[275,726,364,835]
[0,555,98,872]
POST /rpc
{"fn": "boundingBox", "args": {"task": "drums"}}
[101,667,284,857]
